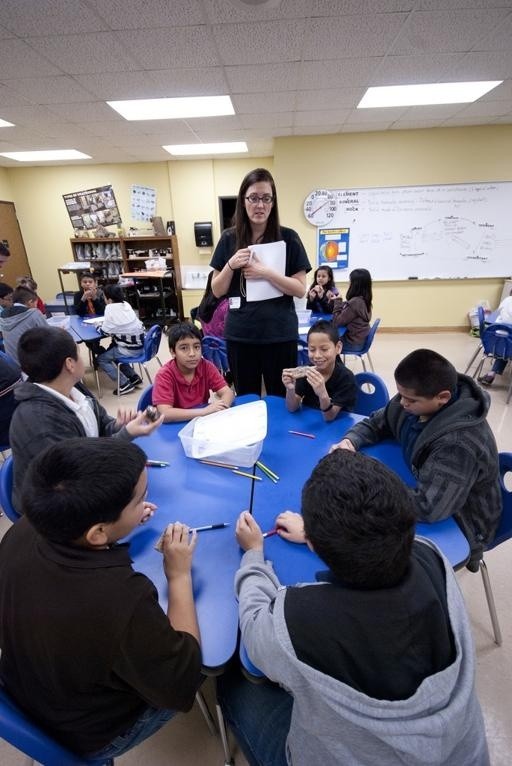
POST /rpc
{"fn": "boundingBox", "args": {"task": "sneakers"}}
[128,374,142,385]
[112,380,136,395]
[476,372,494,386]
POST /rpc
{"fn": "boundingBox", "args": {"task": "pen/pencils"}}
[199,461,238,469]
[263,528,286,538]
[188,523,230,532]
[147,459,170,467]
[289,431,315,439]
[256,461,280,483]
[233,470,263,480]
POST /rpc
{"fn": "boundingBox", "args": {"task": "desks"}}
[116,395,471,678]
[46,314,109,398]
[44,299,74,315]
[298,313,346,344]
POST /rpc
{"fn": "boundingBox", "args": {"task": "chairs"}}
[56,291,74,299]
[341,318,380,391]
[353,373,389,415]
[0,453,21,522]
[464,306,492,374]
[113,324,163,397]
[1,676,215,766]
[473,451,512,645]
[216,705,234,766]
[473,323,512,404]
[202,337,230,376]
[135,384,155,411]
[297,340,311,365]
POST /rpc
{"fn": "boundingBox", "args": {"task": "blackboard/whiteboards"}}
[303,181,512,283]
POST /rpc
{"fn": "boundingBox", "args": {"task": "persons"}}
[0,238,511,766]
[208,167,312,397]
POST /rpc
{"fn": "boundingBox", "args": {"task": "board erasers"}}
[408,277,418,279]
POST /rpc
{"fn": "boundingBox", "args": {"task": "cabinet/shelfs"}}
[121,271,177,326]
[59,265,108,314]
[71,236,183,324]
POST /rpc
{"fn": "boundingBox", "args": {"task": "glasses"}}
[242,192,275,205]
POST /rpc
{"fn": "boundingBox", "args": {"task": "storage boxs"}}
[296,310,312,322]
[178,416,264,466]
[47,316,70,330]
